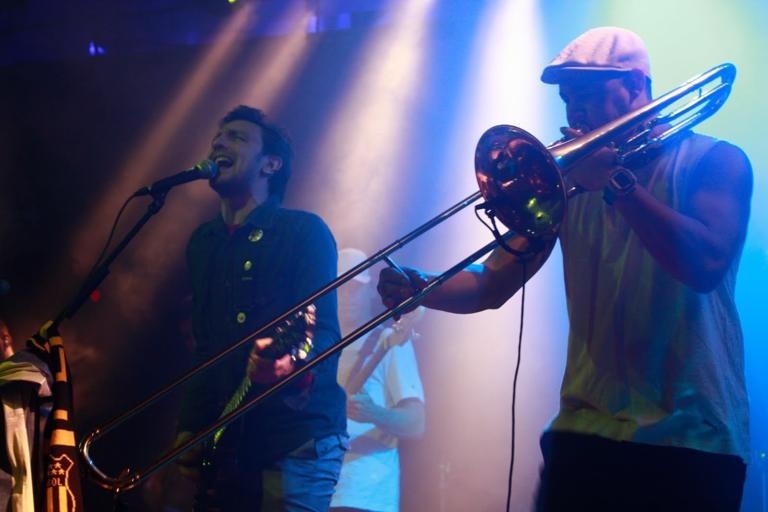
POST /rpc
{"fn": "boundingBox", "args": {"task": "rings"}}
[378,281,386,296]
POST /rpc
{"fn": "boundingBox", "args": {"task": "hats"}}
[542,26,651,84]
[336,249,372,286]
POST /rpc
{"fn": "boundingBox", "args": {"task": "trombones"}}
[78,64,736,494]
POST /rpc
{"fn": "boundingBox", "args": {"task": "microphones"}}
[135,159,217,199]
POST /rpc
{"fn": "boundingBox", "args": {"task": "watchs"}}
[602,168,638,202]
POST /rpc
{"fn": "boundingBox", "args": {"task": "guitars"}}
[140,304,316,511]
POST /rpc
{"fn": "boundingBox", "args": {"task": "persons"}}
[329,247,426,512]
[376,26,754,512]
[163,104,349,512]
